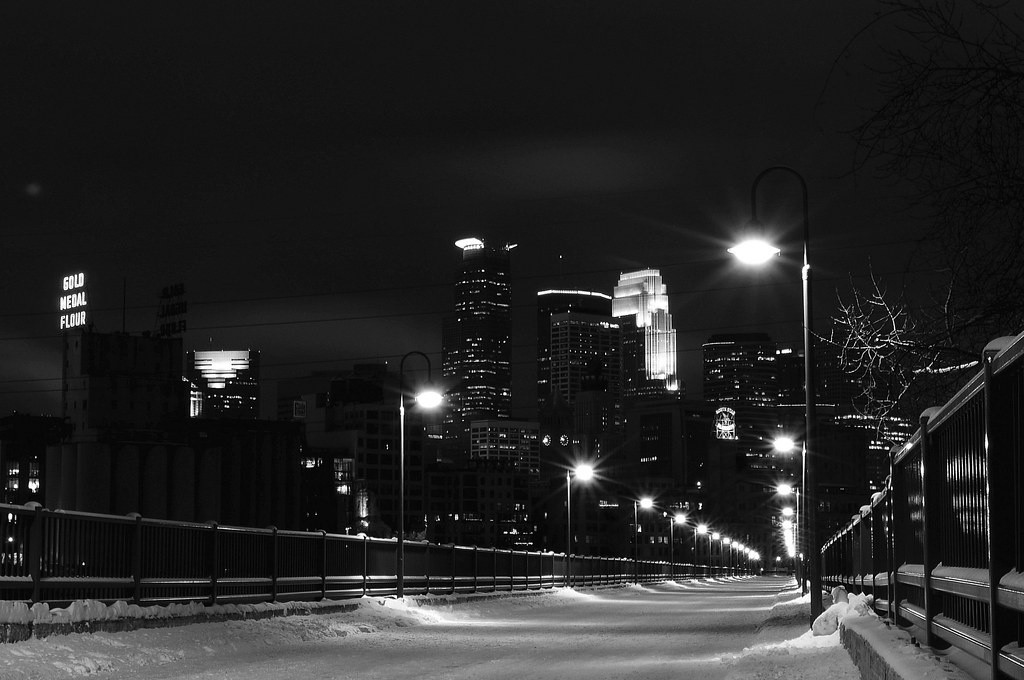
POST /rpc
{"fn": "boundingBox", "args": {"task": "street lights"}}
[565,460,602,586]
[669,511,690,581]
[694,522,762,579]
[728,165,825,630]
[393,350,446,598]
[633,496,657,585]
[773,432,810,597]
